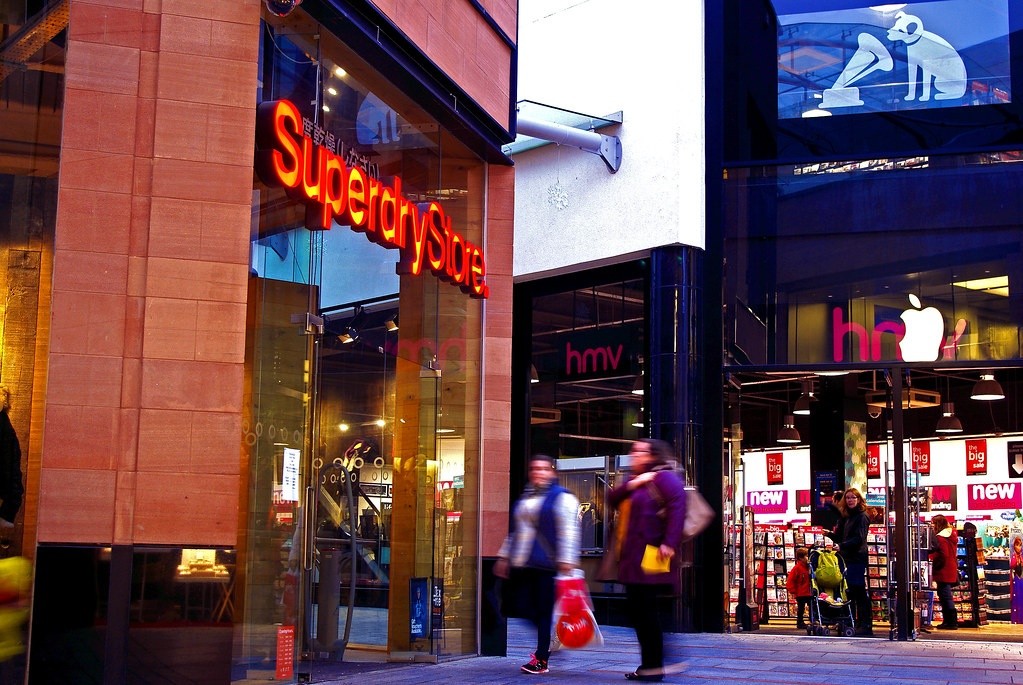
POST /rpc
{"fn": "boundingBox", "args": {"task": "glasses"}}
[845,496,858,499]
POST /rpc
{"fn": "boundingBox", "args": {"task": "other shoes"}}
[797,622,810,628]
[854,619,874,637]
[520,652,549,674]
[625,666,664,680]
[937,623,959,630]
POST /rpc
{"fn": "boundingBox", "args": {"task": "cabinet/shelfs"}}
[753,530,987,626]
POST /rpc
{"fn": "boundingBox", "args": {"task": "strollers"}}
[807,545,857,637]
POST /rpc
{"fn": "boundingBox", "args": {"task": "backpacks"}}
[811,550,842,585]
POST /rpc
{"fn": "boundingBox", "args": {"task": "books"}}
[733,530,986,622]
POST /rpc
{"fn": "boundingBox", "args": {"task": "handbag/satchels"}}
[547,569,604,651]
[649,465,713,542]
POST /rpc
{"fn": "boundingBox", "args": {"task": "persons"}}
[597,436,687,681]
[0,384,24,554]
[271,516,385,605]
[787,488,958,628]
[578,503,596,550]
[494,453,580,674]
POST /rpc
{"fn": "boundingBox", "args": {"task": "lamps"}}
[971,370,1004,400]
[777,382,801,443]
[936,371,963,433]
[530,364,539,383]
[631,398,644,427]
[632,367,644,395]
[793,381,816,415]
[384,312,398,332]
[338,331,353,343]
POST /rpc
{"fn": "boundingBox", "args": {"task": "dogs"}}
[886,11,967,101]
